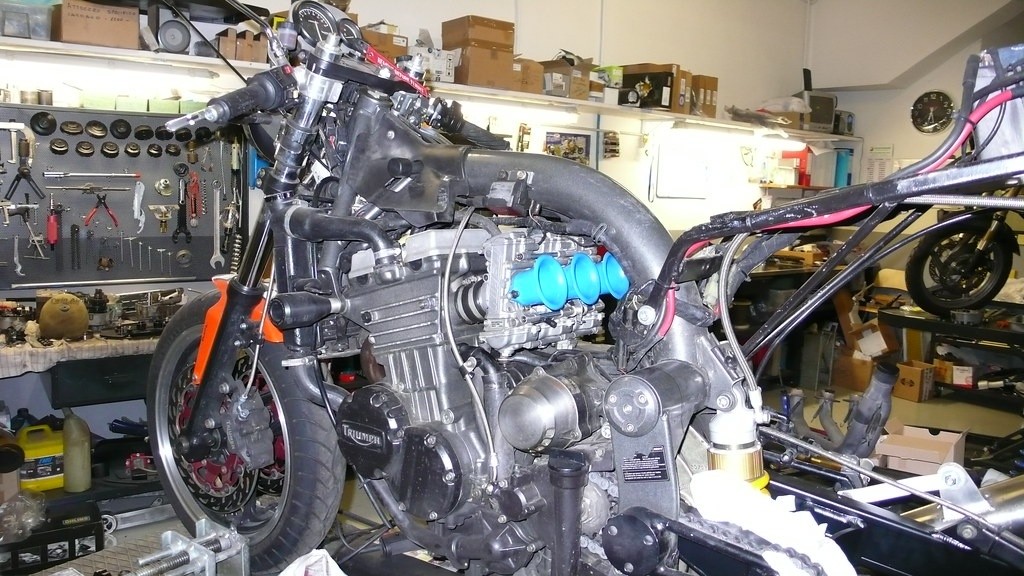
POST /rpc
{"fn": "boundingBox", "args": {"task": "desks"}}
[748,262,848,279]
[877,308,1024,417]
[758,182,833,200]
[0,334,160,379]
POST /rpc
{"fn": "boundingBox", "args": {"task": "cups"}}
[20,90,38,103]
[39,91,53,105]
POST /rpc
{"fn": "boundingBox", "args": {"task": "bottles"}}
[11,406,92,492]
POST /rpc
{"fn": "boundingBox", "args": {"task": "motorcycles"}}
[142,1,1024,576]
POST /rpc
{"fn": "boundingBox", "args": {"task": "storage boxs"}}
[592,65,624,89]
[82,88,207,114]
[0,500,105,576]
[213,27,268,63]
[874,417,971,475]
[454,46,514,91]
[596,87,619,105]
[442,15,515,53]
[659,63,681,113]
[512,53,544,94]
[537,57,600,101]
[621,63,663,74]
[679,70,719,118]
[361,29,408,57]
[51,0,140,50]
[775,250,987,403]
[776,112,801,129]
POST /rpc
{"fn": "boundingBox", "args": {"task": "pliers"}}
[85,194,119,228]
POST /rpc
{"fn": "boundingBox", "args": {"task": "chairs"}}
[855,268,915,323]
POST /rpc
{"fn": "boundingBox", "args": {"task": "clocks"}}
[910,89,956,136]
